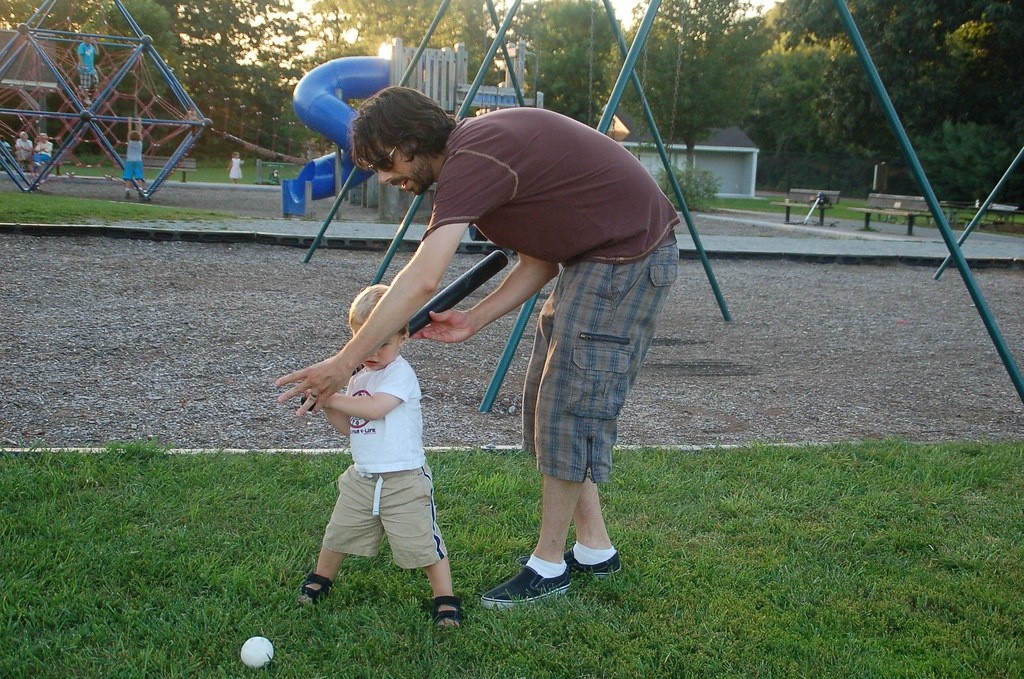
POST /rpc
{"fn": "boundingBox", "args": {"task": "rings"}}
[311,391,319,398]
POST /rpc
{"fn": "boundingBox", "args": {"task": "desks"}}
[942,201,1016,229]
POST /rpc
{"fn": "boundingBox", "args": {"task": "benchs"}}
[113,153,196,183]
[770,187,840,224]
[6,149,71,176]
[848,192,931,235]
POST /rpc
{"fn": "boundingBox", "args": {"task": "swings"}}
[586,0,688,196]
[479,0,543,112]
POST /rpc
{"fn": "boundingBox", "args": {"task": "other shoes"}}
[125,189,130,198]
[139,189,143,197]
[84,98,92,105]
[32,161,41,167]
[78,85,84,94]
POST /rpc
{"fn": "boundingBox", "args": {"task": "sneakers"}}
[515,546,621,576]
[480,566,571,609]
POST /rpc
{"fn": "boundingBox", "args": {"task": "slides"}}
[281,56,392,215]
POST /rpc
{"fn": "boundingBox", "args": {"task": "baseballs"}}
[240,636,274,670]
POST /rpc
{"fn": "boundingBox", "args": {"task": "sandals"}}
[431,596,463,631]
[300,573,333,605]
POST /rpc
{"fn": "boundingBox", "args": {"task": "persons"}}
[0,131,12,162]
[76,36,98,105]
[297,285,464,635]
[16,132,33,172]
[33,133,53,173]
[273,84,682,612]
[122,116,147,199]
[227,151,245,183]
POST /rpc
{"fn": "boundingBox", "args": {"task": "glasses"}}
[367,142,398,175]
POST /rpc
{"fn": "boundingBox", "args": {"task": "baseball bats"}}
[300,249,509,412]
